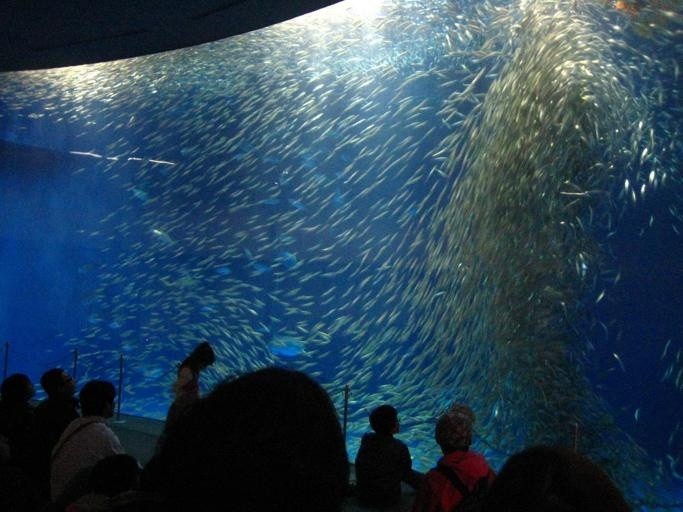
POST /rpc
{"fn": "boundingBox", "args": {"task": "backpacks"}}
[435,464,490,512]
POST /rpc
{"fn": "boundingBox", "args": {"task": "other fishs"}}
[0,0,683,512]
